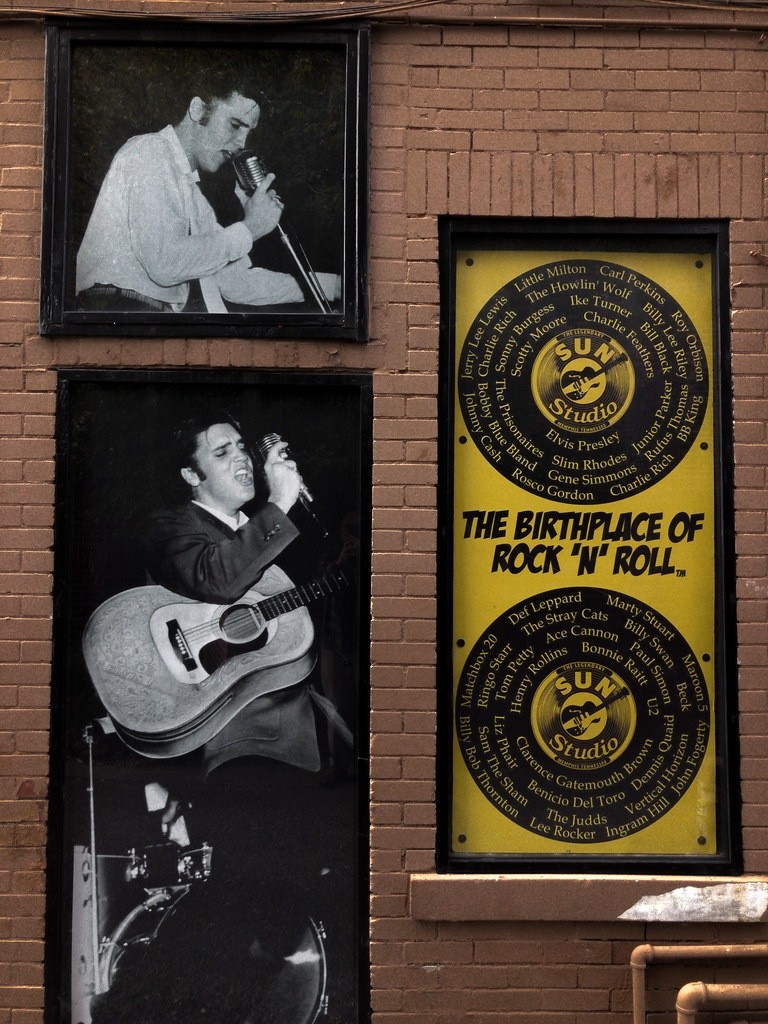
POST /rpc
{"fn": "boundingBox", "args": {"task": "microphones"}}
[232,150,270,193]
[257,432,314,504]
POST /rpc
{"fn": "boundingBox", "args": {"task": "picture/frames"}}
[40,16,368,344]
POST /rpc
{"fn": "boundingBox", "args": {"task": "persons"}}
[90,405,367,1024]
[76,64,341,313]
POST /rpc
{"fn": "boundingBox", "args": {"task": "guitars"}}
[82,558,368,760]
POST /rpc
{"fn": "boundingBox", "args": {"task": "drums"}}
[177,846,213,882]
[98,878,330,1023]
